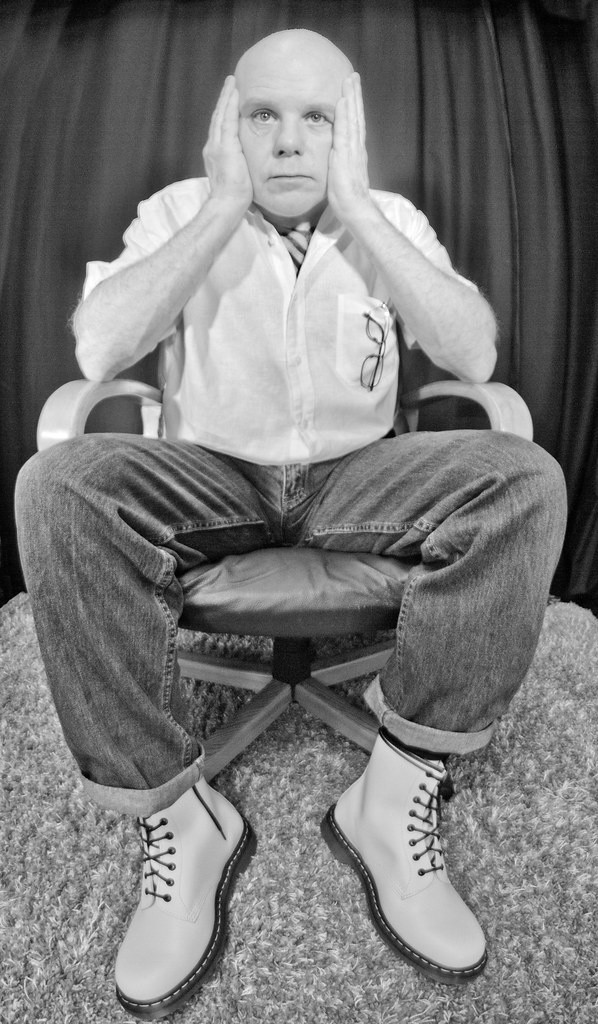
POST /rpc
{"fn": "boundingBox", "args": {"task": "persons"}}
[13,26,569,1021]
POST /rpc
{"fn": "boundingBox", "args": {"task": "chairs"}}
[37,377,535,789]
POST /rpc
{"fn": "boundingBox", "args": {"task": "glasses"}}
[360,307,391,392]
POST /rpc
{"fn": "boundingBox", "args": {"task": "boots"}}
[115,774,257,1017]
[321,726,487,985]
